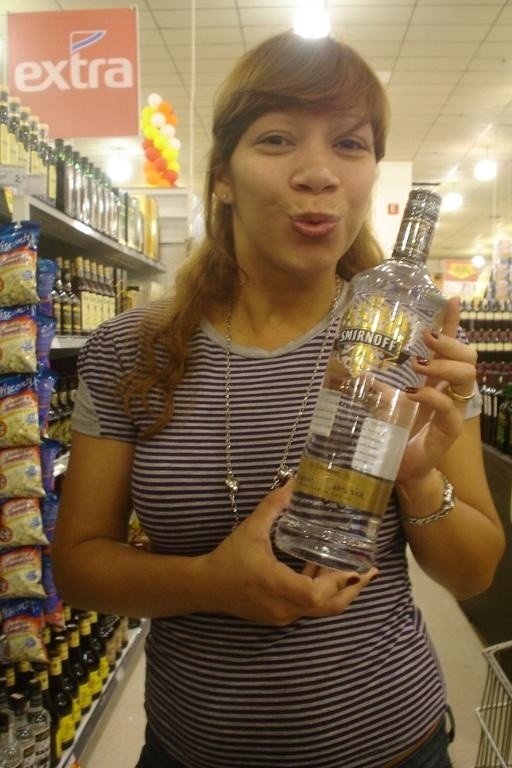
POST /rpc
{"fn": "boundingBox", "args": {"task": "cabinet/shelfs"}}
[0,185,164,768]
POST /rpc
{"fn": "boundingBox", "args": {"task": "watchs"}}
[397,470,457,528]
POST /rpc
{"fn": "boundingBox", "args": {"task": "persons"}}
[49,26,507,766]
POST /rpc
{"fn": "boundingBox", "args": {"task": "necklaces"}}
[224,271,344,532]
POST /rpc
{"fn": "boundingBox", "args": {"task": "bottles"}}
[0,84,163,768]
[274,184,450,579]
[440,295,512,463]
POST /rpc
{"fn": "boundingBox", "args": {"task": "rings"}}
[447,384,476,403]
